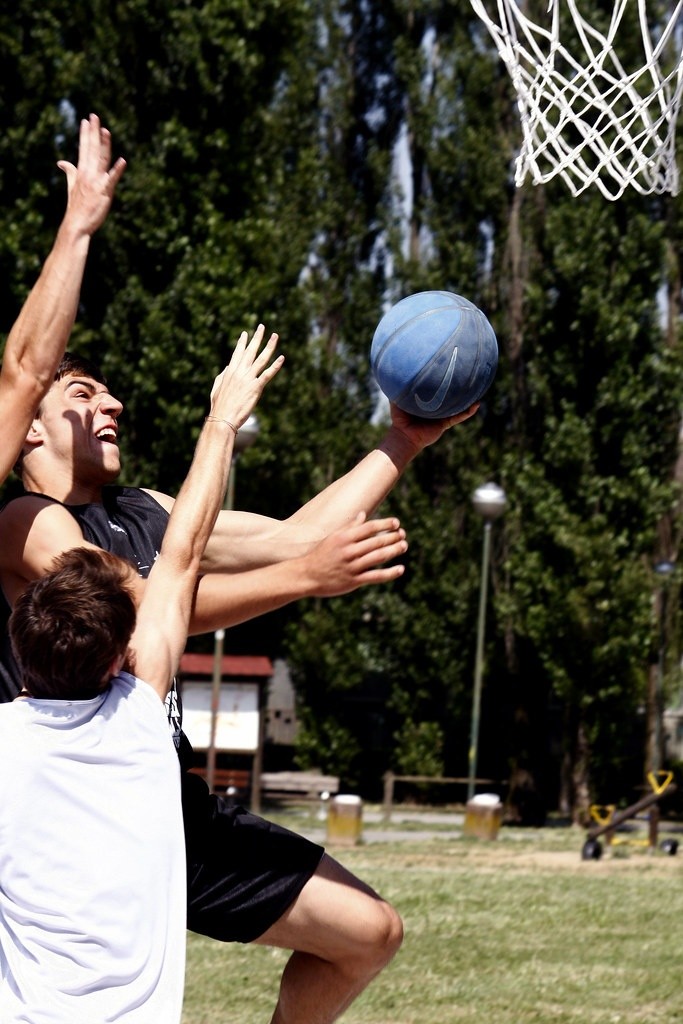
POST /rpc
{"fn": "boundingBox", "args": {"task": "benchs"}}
[186,768,339,819]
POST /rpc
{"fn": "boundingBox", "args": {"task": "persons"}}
[0,361,482,1022]
[4,319,286,1022]
[0,114,126,483]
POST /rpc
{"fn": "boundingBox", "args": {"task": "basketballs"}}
[370,290,499,421]
